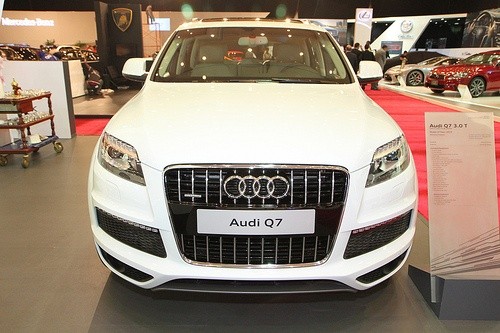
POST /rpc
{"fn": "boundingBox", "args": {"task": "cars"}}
[384,50,448,73]
[425,49,500,98]
[384,56,466,86]
[86,15,421,300]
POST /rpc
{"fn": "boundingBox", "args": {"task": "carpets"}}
[74,118,111,136]
[360,83,500,229]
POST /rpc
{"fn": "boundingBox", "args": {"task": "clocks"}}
[401,20,413,33]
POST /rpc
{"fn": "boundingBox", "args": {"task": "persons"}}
[263,48,271,59]
[345,45,358,74]
[371,45,388,90]
[462,21,496,48]
[352,43,363,56]
[246,48,257,58]
[400,51,408,64]
[359,45,376,90]
[84,58,103,94]
[39,45,65,61]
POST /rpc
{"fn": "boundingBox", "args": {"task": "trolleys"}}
[0,79,65,169]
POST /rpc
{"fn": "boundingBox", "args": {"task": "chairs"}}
[191,42,319,78]
[491,58,498,65]
